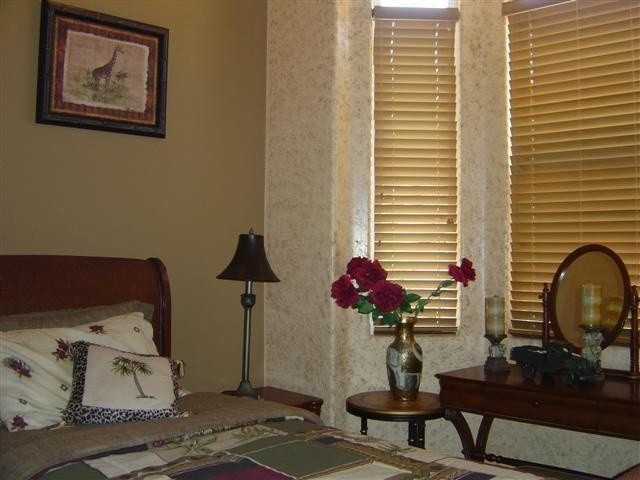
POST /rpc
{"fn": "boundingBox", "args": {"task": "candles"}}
[485,296,505,336]
[580,284,602,326]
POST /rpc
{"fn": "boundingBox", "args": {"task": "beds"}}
[1,251,543,480]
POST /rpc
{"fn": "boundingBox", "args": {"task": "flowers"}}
[331,256,476,324]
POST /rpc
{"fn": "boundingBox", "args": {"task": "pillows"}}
[0,301,191,433]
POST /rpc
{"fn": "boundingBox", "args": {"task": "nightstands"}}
[223,385,324,419]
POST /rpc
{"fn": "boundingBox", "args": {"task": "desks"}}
[434,363,639,480]
[345,391,446,450]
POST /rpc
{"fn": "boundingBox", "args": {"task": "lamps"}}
[214,227,281,400]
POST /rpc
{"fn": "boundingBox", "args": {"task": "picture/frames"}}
[35,1,169,140]
[387,316,424,402]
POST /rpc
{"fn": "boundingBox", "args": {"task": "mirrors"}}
[549,243,633,355]
